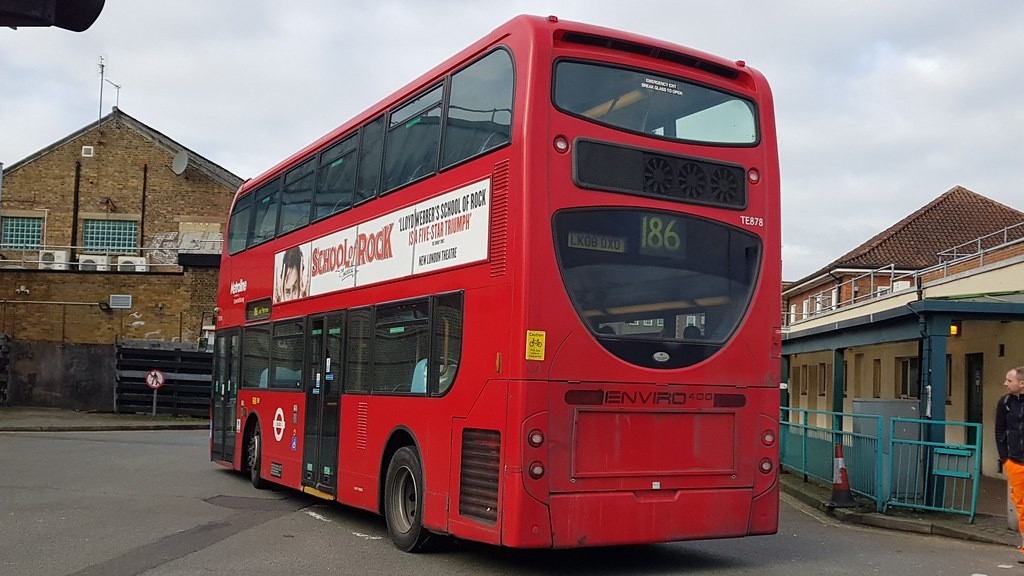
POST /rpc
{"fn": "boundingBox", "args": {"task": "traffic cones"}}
[829,443,856,509]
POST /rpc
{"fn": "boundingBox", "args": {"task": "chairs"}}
[250,130,507,246]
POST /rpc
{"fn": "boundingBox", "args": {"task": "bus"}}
[196,13,784,551]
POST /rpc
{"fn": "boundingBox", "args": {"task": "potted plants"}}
[118,255,149,272]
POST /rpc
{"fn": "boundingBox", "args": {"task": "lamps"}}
[99,198,116,214]
[950,322,962,335]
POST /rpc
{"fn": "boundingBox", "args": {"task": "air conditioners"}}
[79,254,112,270]
[38,250,69,269]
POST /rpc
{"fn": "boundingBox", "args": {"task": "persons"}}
[994,367,1024,564]
[275,248,309,303]
